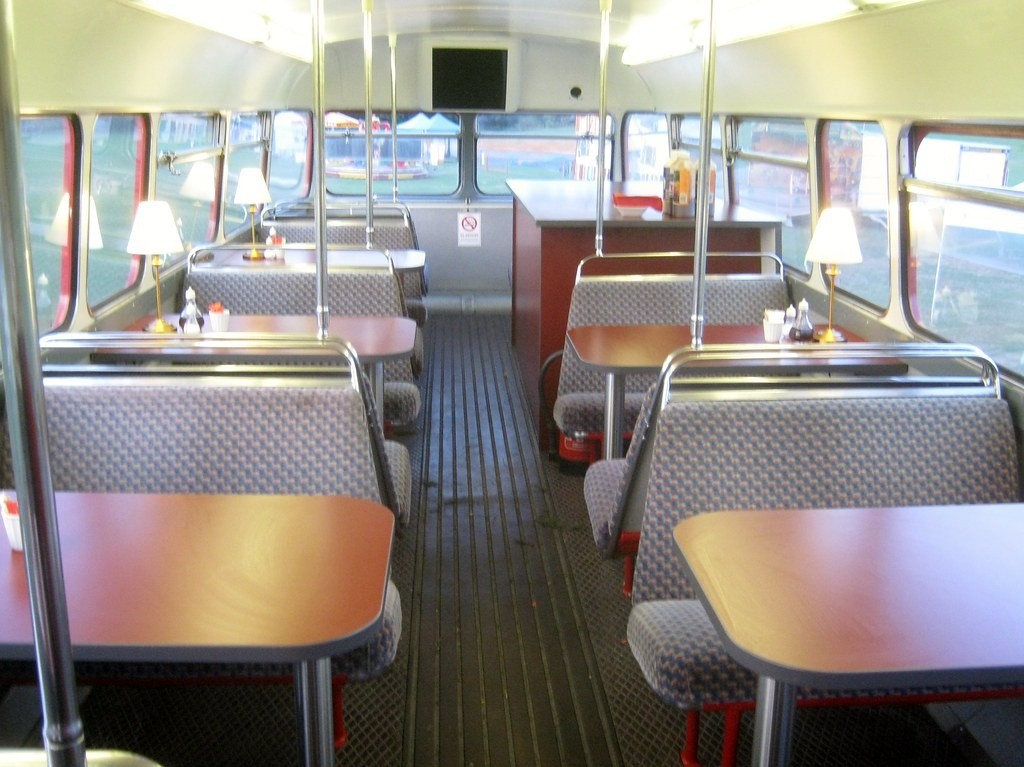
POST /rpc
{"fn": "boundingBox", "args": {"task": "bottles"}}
[662,149,696,218]
[784,303,797,339]
[178,285,205,329]
[789,297,814,341]
[182,303,201,337]
[263,226,286,261]
[695,155,717,218]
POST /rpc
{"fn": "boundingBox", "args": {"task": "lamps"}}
[179,163,217,240]
[127,198,184,333]
[46,193,102,251]
[233,165,271,261]
[805,208,862,339]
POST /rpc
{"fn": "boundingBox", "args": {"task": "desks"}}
[183,247,426,317]
[566,322,907,461]
[90,310,416,440]
[1,493,395,762]
[668,503,1021,765]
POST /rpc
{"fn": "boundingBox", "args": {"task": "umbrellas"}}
[396,112,461,140]
[325,111,360,132]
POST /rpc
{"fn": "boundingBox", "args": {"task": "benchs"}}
[1,198,429,766]
[540,243,1024,767]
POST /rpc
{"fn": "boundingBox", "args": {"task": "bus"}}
[475,113,668,182]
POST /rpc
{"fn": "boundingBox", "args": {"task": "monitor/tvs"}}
[432,47,508,113]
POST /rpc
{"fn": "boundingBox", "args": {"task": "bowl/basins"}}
[614,203,648,218]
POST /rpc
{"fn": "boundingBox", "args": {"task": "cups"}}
[2,502,25,551]
[275,249,285,260]
[208,308,230,332]
[763,319,784,343]
